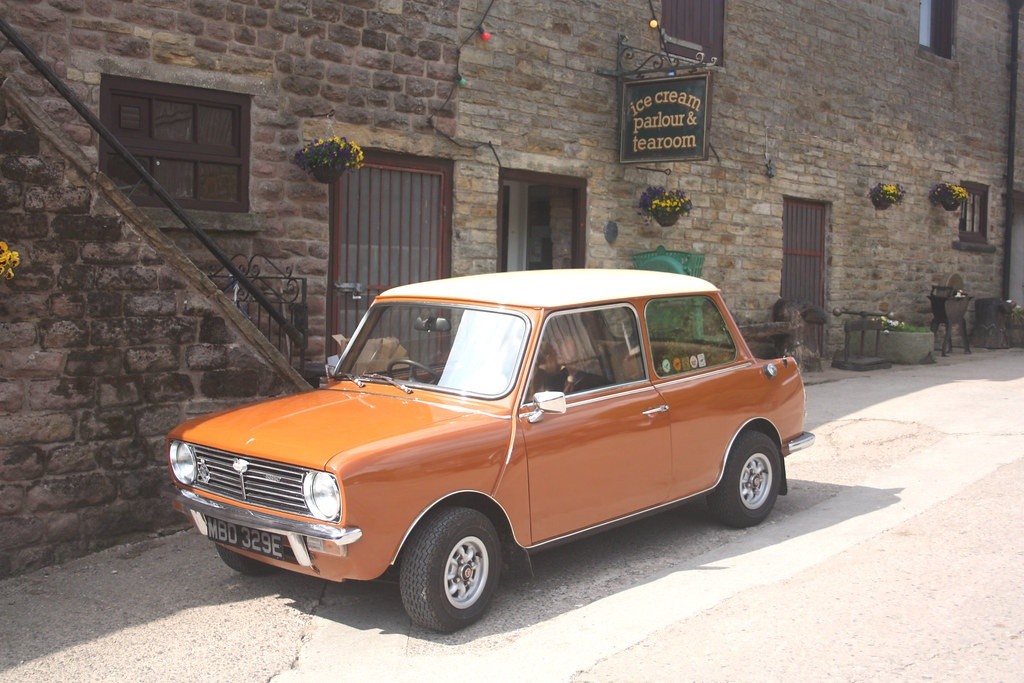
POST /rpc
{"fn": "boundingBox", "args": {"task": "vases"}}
[311,168,344,183]
[876,332,935,364]
[654,211,680,227]
[943,201,960,211]
[873,200,892,210]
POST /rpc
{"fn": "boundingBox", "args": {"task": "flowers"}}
[0,241,21,281]
[929,182,969,206]
[871,312,932,334]
[637,185,693,226]
[294,137,364,174]
[867,182,906,206]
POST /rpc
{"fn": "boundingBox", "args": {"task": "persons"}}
[537,319,604,395]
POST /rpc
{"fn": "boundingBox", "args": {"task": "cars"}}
[166,269,815,635]
